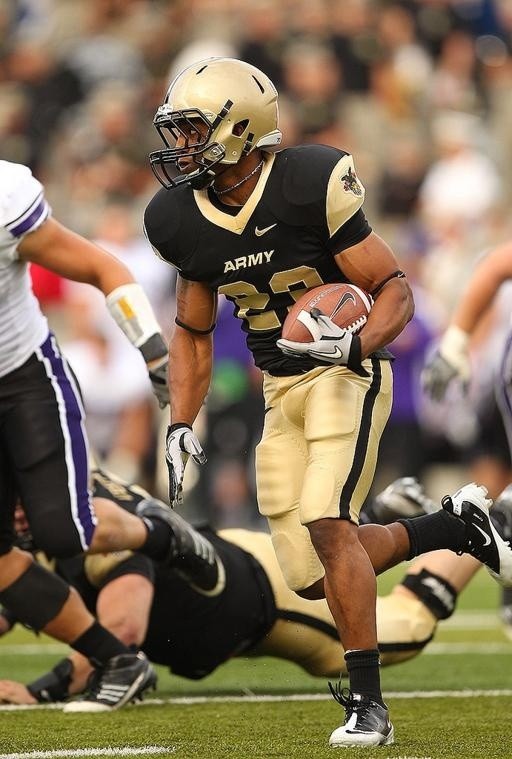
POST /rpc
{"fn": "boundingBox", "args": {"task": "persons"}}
[0,460,512,532]
[143,57,512,749]
[0,159,212,714]
[0,1,512,634]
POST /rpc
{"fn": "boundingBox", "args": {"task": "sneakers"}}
[137,497,227,597]
[488,483,512,540]
[329,673,395,747]
[373,477,435,521]
[439,483,512,590]
[62,643,156,713]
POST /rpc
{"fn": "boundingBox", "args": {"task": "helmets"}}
[150,57,281,195]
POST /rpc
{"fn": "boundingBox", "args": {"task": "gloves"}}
[418,323,473,402]
[164,423,208,509]
[278,308,371,378]
[137,334,211,408]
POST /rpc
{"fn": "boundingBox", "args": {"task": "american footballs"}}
[281,282,373,358]
